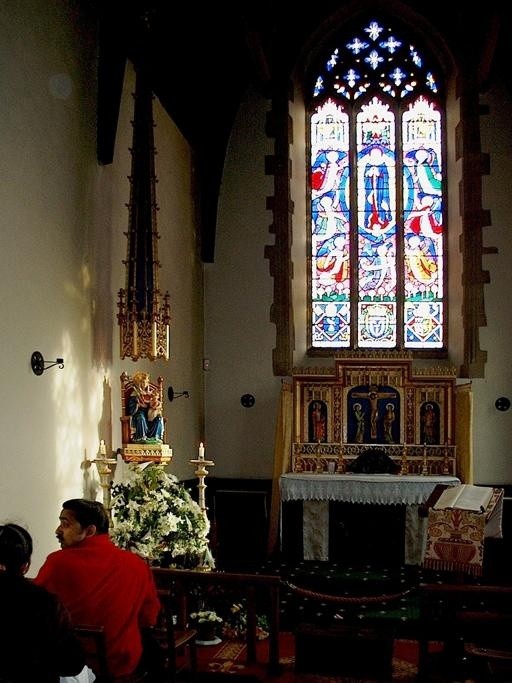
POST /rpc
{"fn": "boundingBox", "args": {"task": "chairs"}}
[24,576,195,680]
[443,632,510,682]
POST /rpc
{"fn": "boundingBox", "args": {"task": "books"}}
[434,482,495,513]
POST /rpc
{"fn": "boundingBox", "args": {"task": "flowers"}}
[223,602,268,639]
[107,461,218,572]
[189,610,223,623]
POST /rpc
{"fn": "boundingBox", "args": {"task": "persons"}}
[127,370,165,442]
[353,390,396,436]
[383,402,396,443]
[32,497,161,682]
[362,144,393,228]
[311,402,327,441]
[421,404,437,443]
[1,521,100,682]
[352,402,366,443]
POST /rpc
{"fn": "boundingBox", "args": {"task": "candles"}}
[100,439,106,452]
[199,442,204,457]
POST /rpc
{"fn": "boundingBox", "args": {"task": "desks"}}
[277,472,463,566]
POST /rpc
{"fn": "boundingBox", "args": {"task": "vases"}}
[189,620,214,642]
